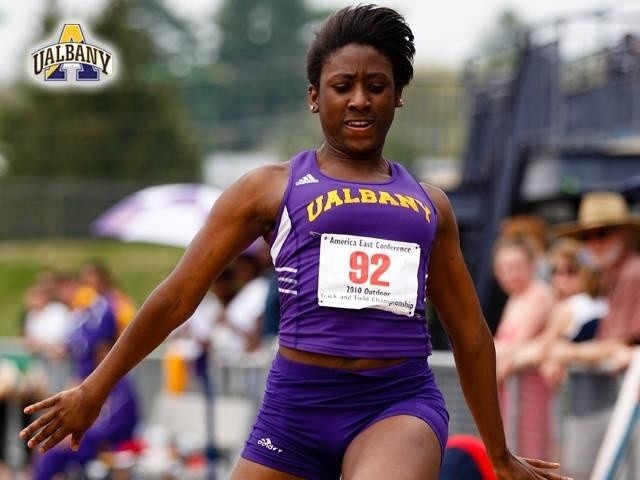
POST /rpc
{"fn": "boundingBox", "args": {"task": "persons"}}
[19,5,572,479]
[22,251,280,480]
[493,192,639,384]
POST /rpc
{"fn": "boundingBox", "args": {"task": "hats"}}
[555,190,640,239]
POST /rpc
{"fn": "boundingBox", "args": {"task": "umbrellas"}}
[91,184,268,250]
